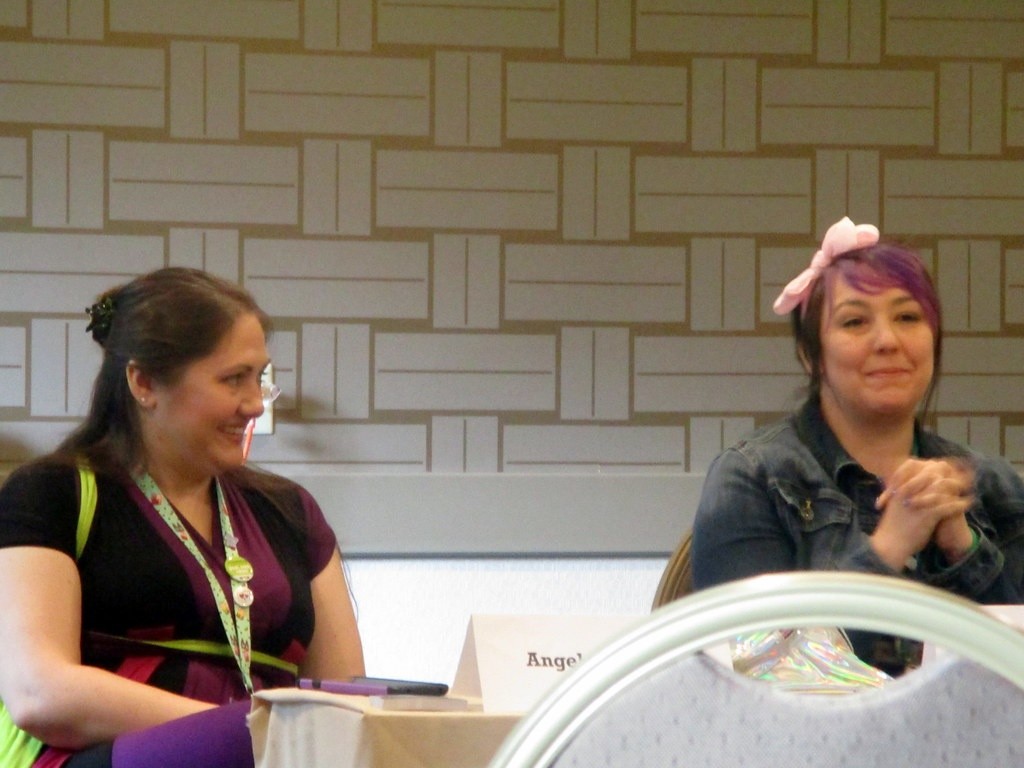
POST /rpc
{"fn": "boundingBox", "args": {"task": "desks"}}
[247,687,526,767]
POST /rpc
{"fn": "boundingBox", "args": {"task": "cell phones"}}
[296,675,448,698]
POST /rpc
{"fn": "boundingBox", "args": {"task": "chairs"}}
[493,538,1024,768]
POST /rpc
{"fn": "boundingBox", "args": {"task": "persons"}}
[0,265,366,768]
[692,217,1024,678]
